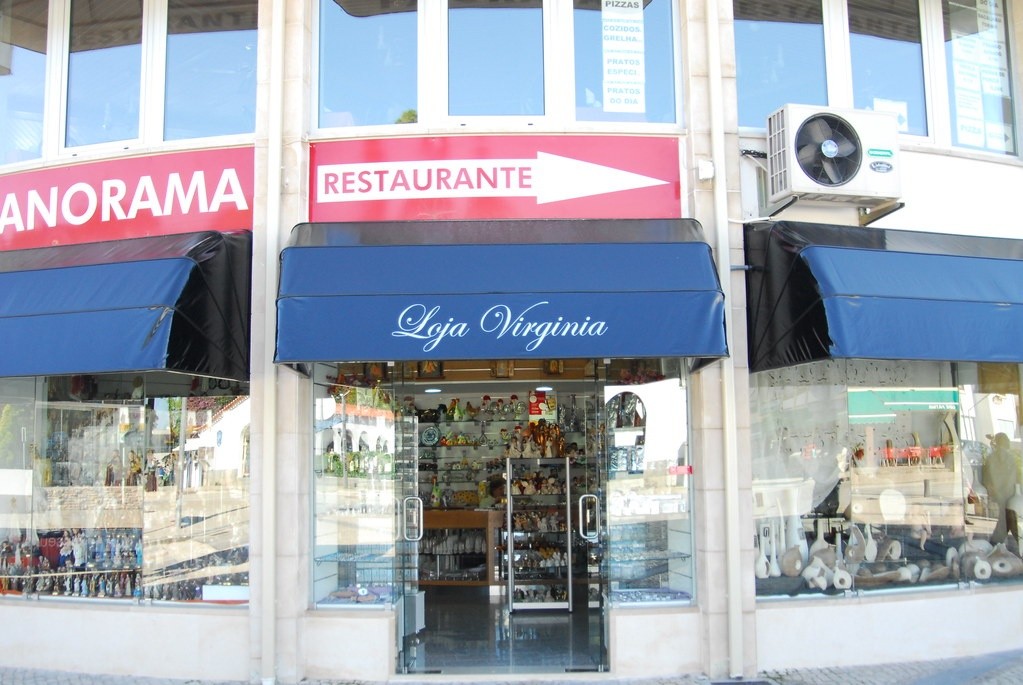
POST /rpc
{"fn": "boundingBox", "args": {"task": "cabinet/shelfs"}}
[393,380,607,587]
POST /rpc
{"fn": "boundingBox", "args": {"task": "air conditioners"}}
[765,102,902,204]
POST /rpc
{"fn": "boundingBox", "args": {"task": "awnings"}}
[0,228,251,383]
[274,218,730,375]
[743,220,1023,374]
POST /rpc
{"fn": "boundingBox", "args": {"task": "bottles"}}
[755,488,843,579]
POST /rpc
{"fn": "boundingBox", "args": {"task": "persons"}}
[945,500,974,541]
[985,433,1017,547]
[908,503,932,551]
[0,449,250,600]
